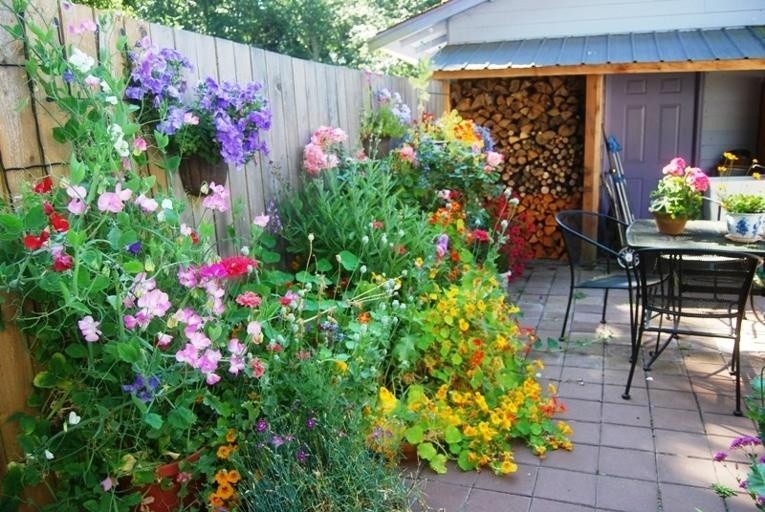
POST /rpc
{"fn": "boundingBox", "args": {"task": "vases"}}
[652,214,687,234]
[728,213,763,239]
[128,446,204,511]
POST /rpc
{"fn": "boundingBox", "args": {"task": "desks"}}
[626,218,765,371]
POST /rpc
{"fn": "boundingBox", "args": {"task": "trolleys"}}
[599,123,645,273]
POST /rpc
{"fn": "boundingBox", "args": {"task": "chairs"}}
[623,245,763,417]
[555,210,678,342]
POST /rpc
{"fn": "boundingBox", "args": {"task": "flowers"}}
[649,157,710,219]
[716,152,765,213]
